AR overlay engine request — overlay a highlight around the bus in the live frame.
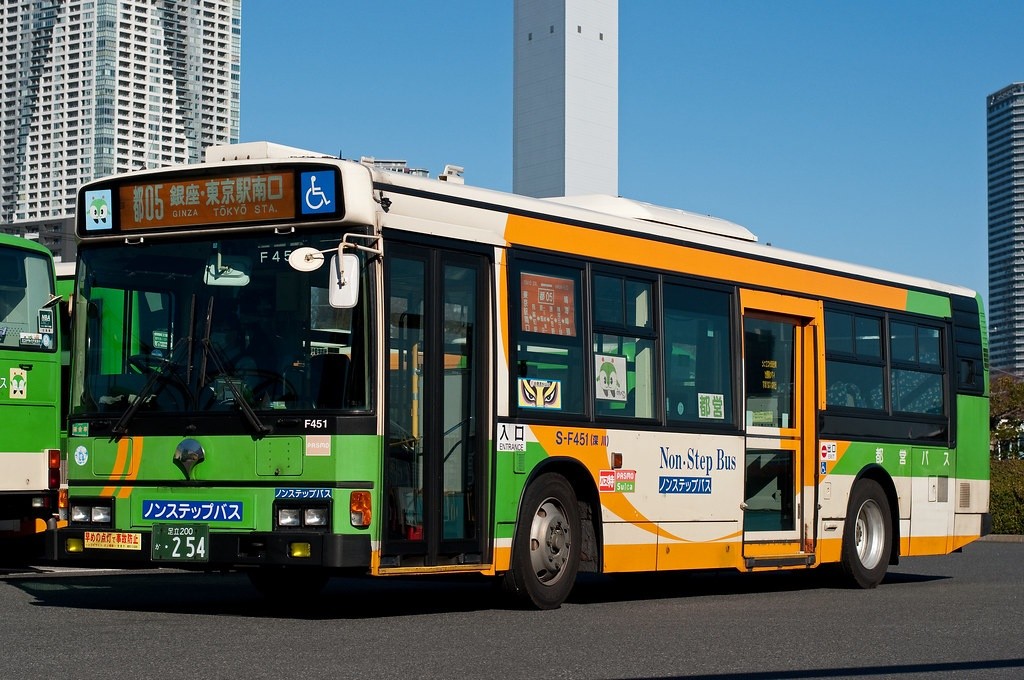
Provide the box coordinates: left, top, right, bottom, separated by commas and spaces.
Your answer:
0, 231, 63, 538
58, 141, 993, 610
